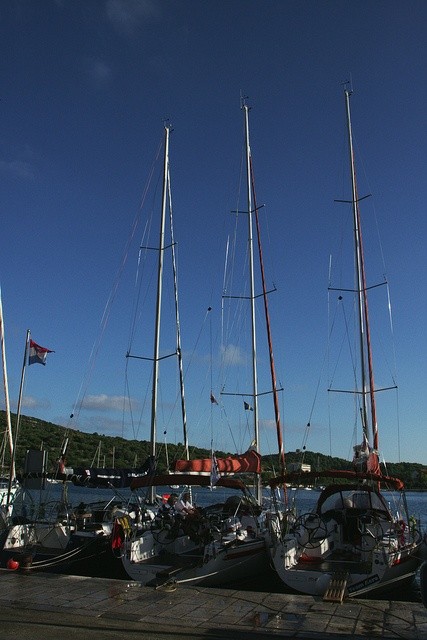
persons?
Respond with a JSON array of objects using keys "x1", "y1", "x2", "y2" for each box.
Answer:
[
  {"x1": 177, "y1": 492, "x2": 194, "y2": 516},
  {"x1": 156, "y1": 495, "x2": 175, "y2": 525}
]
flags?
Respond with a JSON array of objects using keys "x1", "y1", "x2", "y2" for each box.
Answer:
[{"x1": 28, "y1": 341, "x2": 54, "y2": 367}]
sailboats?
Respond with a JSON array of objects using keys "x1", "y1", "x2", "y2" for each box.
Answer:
[
  {"x1": 269, "y1": 71, "x2": 427, "y2": 598},
  {"x1": 0, "y1": 295, "x2": 25, "y2": 517},
  {"x1": 112, "y1": 90, "x2": 298, "y2": 586},
  {"x1": 0, "y1": 117, "x2": 193, "y2": 570}
]
